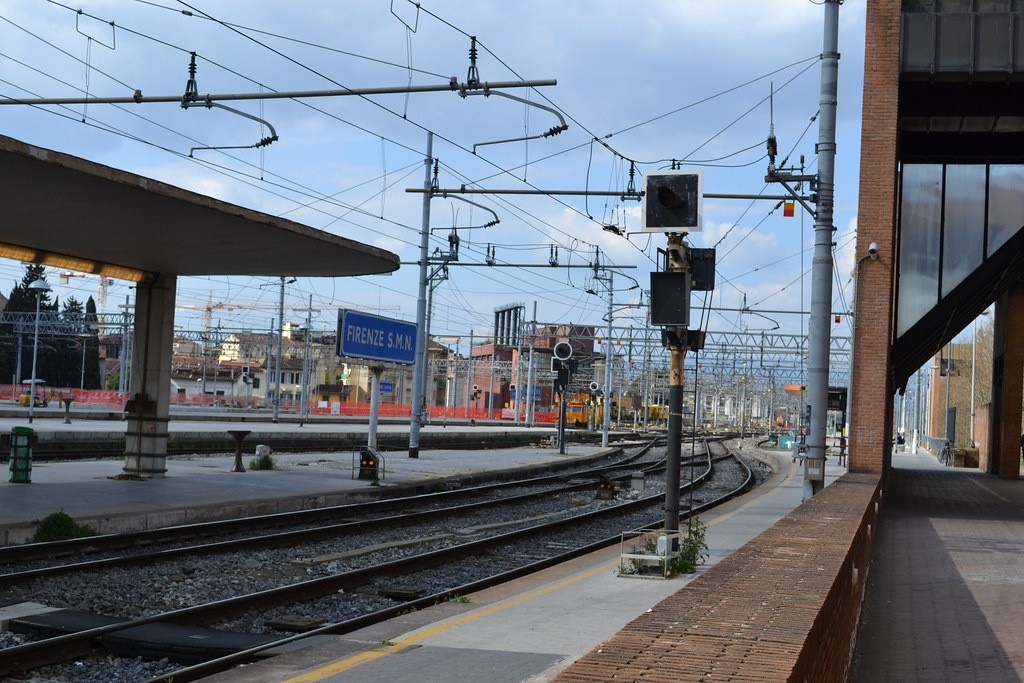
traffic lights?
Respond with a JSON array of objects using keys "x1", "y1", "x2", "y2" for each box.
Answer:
[{"x1": 645, "y1": 172, "x2": 702, "y2": 231}]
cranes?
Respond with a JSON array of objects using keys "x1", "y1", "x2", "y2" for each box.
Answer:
[
  {"x1": 60, "y1": 269, "x2": 116, "y2": 335},
  {"x1": 179, "y1": 288, "x2": 400, "y2": 351}
]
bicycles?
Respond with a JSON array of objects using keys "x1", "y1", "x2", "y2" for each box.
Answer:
[{"x1": 937, "y1": 439, "x2": 953, "y2": 467}]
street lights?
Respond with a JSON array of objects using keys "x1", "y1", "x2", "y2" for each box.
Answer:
[{"x1": 26, "y1": 271, "x2": 51, "y2": 422}]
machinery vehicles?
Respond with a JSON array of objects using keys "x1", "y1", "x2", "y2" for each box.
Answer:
[{"x1": 19, "y1": 378, "x2": 61, "y2": 407}]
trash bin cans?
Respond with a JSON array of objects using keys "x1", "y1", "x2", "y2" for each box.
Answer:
[{"x1": 8, "y1": 426, "x2": 39, "y2": 484}]
